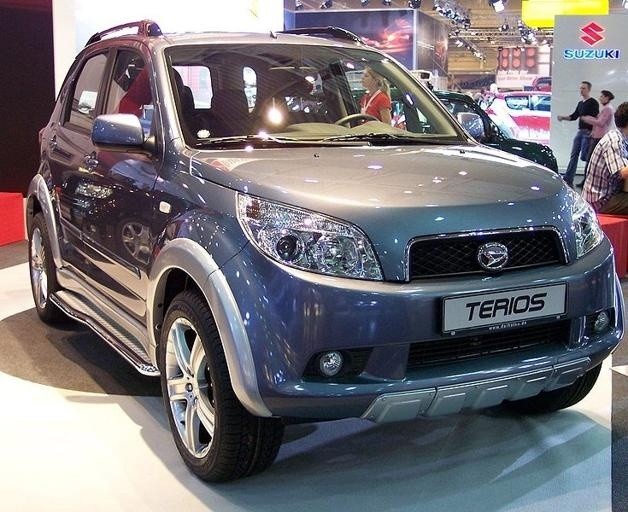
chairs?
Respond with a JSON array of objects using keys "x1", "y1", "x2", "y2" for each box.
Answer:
[{"x1": 175, "y1": 68, "x2": 329, "y2": 135}]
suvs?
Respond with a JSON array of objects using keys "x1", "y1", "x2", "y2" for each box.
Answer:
[{"x1": 23, "y1": 15, "x2": 628, "y2": 485}]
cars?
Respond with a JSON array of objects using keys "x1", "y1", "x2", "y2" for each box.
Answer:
[
  {"x1": 477, "y1": 88, "x2": 552, "y2": 144},
  {"x1": 346, "y1": 84, "x2": 561, "y2": 175}
]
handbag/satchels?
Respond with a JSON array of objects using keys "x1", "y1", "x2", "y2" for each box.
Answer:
[{"x1": 580, "y1": 135, "x2": 590, "y2": 161}]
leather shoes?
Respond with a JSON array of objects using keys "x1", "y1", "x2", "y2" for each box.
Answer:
[{"x1": 576, "y1": 179, "x2": 585, "y2": 188}]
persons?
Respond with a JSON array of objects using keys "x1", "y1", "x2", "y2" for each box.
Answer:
[
  {"x1": 360, "y1": 66, "x2": 391, "y2": 127},
  {"x1": 557, "y1": 81, "x2": 615, "y2": 187},
  {"x1": 472, "y1": 87, "x2": 486, "y2": 102},
  {"x1": 581, "y1": 102, "x2": 628, "y2": 214}
]
